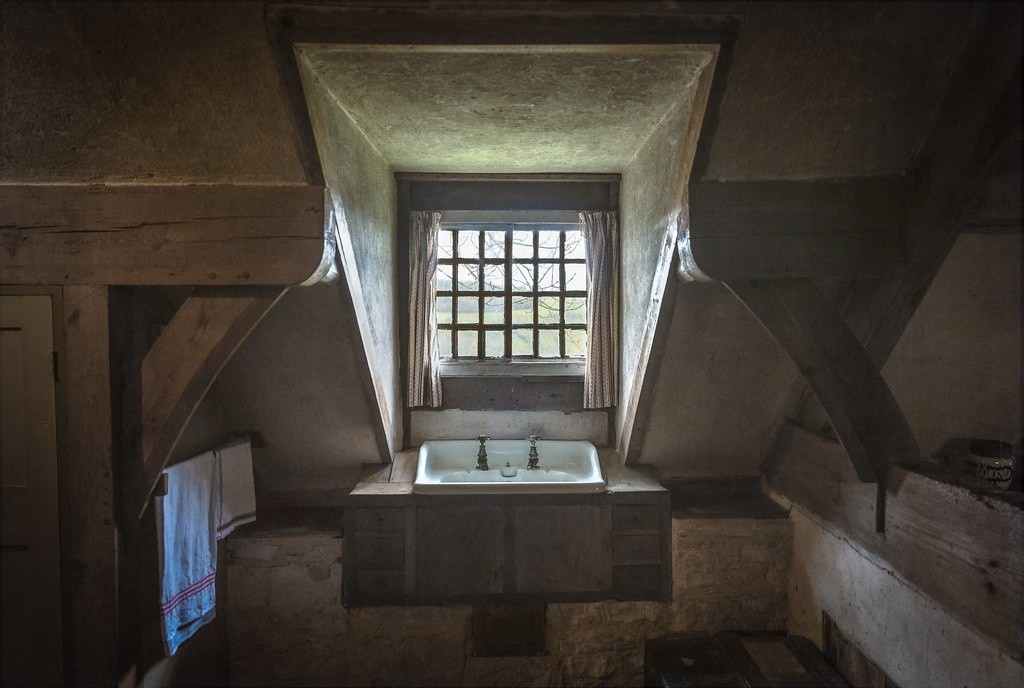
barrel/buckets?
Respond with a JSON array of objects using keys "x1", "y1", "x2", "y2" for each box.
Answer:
[{"x1": 965, "y1": 439, "x2": 1016, "y2": 492}]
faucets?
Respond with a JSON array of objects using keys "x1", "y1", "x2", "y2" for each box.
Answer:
[
  {"x1": 476, "y1": 435, "x2": 490, "y2": 470},
  {"x1": 526, "y1": 434, "x2": 541, "y2": 469}
]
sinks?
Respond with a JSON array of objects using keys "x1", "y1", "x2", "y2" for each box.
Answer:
[{"x1": 413, "y1": 438, "x2": 606, "y2": 494}]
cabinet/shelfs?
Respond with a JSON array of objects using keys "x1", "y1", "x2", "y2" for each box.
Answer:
[{"x1": 338, "y1": 464, "x2": 675, "y2": 607}]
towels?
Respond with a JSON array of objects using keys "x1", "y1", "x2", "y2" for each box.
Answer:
[
  {"x1": 154, "y1": 449, "x2": 218, "y2": 658},
  {"x1": 212, "y1": 437, "x2": 257, "y2": 541}
]
